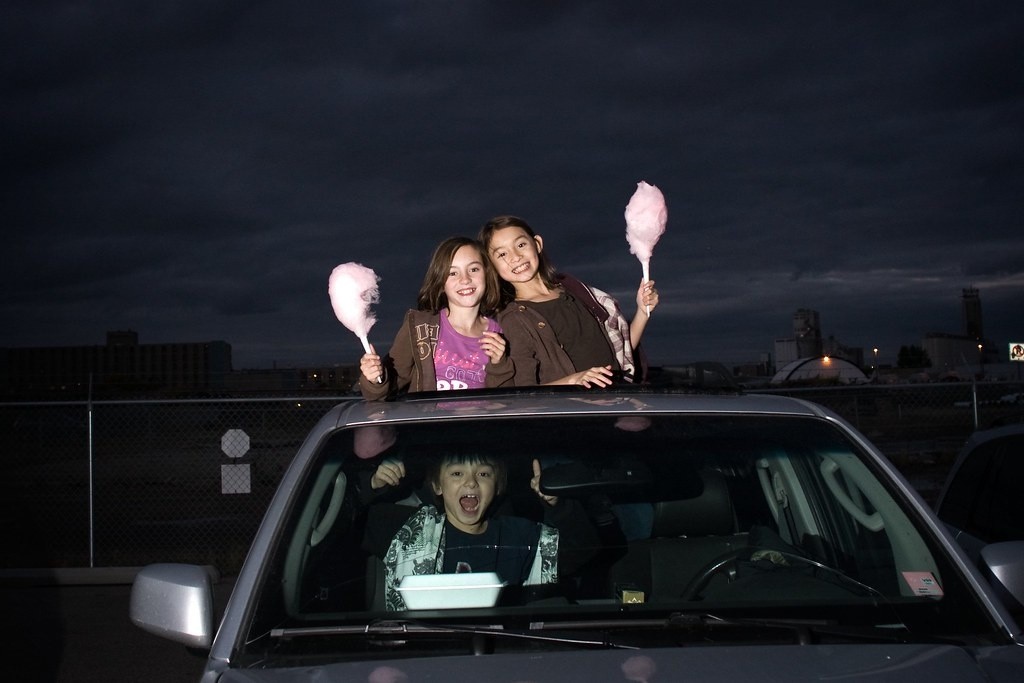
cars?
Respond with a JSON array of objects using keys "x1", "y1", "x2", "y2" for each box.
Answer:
[
  {"x1": 931, "y1": 418, "x2": 1023, "y2": 545},
  {"x1": 124, "y1": 381, "x2": 1024, "y2": 683}
]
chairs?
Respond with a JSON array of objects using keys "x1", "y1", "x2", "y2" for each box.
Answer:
[
  {"x1": 368, "y1": 449, "x2": 515, "y2": 611},
  {"x1": 604, "y1": 477, "x2": 829, "y2": 601}
]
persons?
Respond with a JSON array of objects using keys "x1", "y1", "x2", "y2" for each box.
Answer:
[
  {"x1": 478, "y1": 216, "x2": 656, "y2": 484},
  {"x1": 350, "y1": 432, "x2": 602, "y2": 615},
  {"x1": 357, "y1": 236, "x2": 517, "y2": 458}
]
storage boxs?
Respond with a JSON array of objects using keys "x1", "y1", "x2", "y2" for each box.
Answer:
[{"x1": 389, "y1": 574, "x2": 508, "y2": 610}]
